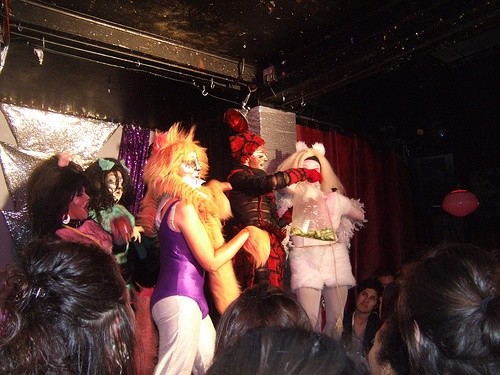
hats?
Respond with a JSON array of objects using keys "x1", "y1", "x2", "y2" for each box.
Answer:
[{"x1": 229, "y1": 129, "x2": 265, "y2": 165}]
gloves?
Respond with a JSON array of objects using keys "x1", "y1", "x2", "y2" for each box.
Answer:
[{"x1": 283, "y1": 168, "x2": 323, "y2": 186}]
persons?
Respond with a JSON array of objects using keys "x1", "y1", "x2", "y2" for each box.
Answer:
[
  {"x1": 0, "y1": 124, "x2": 500, "y2": 375},
  {"x1": 274, "y1": 141, "x2": 368, "y2": 339}
]
